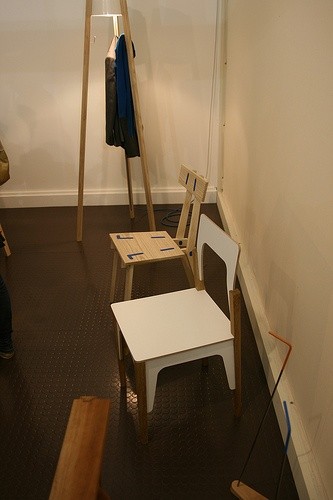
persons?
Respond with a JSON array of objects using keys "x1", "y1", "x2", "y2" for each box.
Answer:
[{"x1": 0, "y1": 140, "x2": 19, "y2": 358}]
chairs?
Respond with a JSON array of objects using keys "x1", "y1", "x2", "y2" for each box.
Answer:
[
  {"x1": 107, "y1": 212, "x2": 244, "y2": 440},
  {"x1": 105, "y1": 163, "x2": 209, "y2": 302}
]
